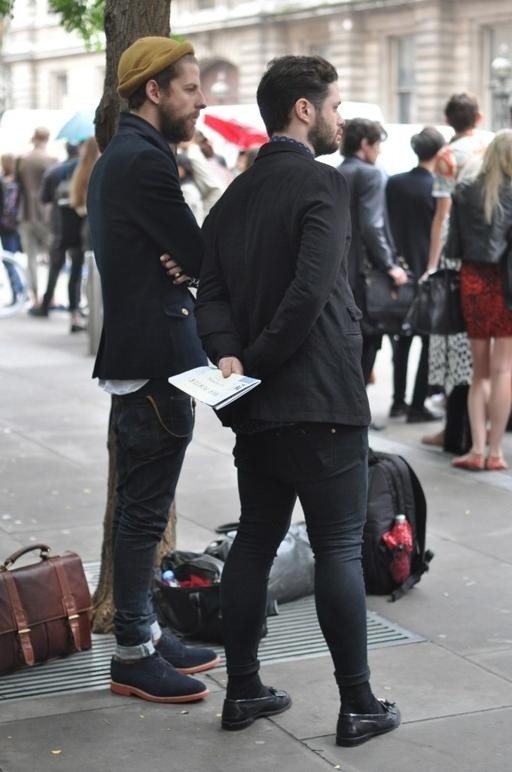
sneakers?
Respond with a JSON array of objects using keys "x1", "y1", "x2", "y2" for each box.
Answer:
[
  {"x1": 110, "y1": 633, "x2": 220, "y2": 704},
  {"x1": 452, "y1": 453, "x2": 508, "y2": 469}
]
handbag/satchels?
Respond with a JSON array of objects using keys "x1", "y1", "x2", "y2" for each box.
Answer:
[
  {"x1": 1, "y1": 544, "x2": 93, "y2": 677},
  {"x1": 443, "y1": 386, "x2": 472, "y2": 454},
  {"x1": 152, "y1": 521, "x2": 315, "y2": 643},
  {"x1": 361, "y1": 452, "x2": 427, "y2": 595},
  {"x1": 356, "y1": 270, "x2": 466, "y2": 334}
]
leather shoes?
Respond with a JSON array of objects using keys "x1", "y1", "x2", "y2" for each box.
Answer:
[
  {"x1": 336, "y1": 700, "x2": 400, "y2": 747},
  {"x1": 221, "y1": 688, "x2": 291, "y2": 730}
]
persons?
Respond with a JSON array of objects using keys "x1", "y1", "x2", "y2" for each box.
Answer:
[
  {"x1": 194, "y1": 57, "x2": 400, "y2": 748},
  {"x1": 0, "y1": 92, "x2": 261, "y2": 334},
  {"x1": 335, "y1": 92, "x2": 512, "y2": 471},
  {"x1": 87, "y1": 37, "x2": 219, "y2": 705}
]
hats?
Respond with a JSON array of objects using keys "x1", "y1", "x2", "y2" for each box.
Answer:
[{"x1": 116, "y1": 36, "x2": 193, "y2": 98}]
